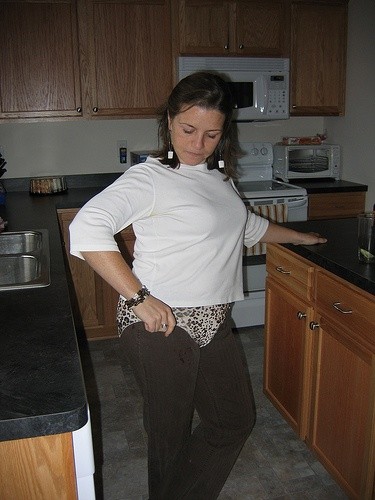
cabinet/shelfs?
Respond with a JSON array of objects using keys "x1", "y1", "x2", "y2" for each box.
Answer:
[
  {"x1": 308, "y1": 192, "x2": 365, "y2": 221},
  {"x1": 57, "y1": 208, "x2": 136, "y2": 342},
  {"x1": 0, "y1": 0, "x2": 349, "y2": 124},
  {"x1": 262, "y1": 242, "x2": 375, "y2": 500}
]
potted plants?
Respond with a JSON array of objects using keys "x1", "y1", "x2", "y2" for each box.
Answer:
[{"x1": 0, "y1": 152, "x2": 8, "y2": 205}]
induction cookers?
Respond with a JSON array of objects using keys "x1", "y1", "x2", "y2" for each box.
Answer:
[{"x1": 230, "y1": 141, "x2": 307, "y2": 200}]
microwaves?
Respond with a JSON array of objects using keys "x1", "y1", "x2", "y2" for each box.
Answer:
[{"x1": 176, "y1": 55, "x2": 290, "y2": 122}]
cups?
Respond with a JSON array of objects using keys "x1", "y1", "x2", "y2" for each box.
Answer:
[{"x1": 357, "y1": 213, "x2": 375, "y2": 264}]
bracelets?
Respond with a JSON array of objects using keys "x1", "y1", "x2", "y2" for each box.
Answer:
[{"x1": 124, "y1": 285, "x2": 151, "y2": 310}]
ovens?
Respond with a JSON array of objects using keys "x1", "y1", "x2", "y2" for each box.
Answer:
[
  {"x1": 272, "y1": 142, "x2": 341, "y2": 182},
  {"x1": 230, "y1": 195, "x2": 310, "y2": 330}
]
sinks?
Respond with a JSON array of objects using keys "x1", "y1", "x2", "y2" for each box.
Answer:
[
  {"x1": 0, "y1": 228, "x2": 50, "y2": 256},
  {"x1": 0, "y1": 254, "x2": 50, "y2": 291}
]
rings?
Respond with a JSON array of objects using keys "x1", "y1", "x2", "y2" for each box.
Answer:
[{"x1": 160, "y1": 323, "x2": 169, "y2": 329}]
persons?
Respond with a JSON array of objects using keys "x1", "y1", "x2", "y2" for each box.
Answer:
[{"x1": 68, "y1": 70, "x2": 327, "y2": 500}]
